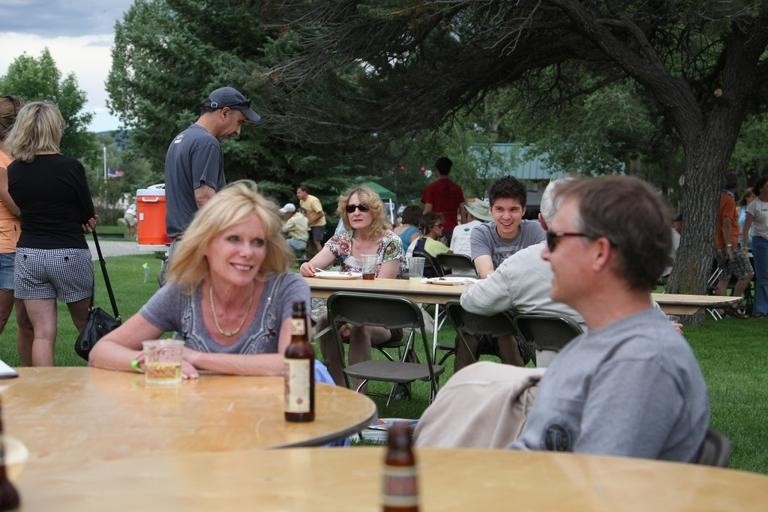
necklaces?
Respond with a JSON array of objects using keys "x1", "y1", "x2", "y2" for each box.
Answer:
[{"x1": 206, "y1": 281, "x2": 255, "y2": 339}]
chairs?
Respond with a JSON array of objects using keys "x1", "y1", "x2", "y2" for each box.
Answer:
[
  {"x1": 341, "y1": 273, "x2": 427, "y2": 409},
  {"x1": 327, "y1": 292, "x2": 445, "y2": 411},
  {"x1": 404, "y1": 250, "x2": 581, "y2": 369},
  {"x1": 708, "y1": 262, "x2": 731, "y2": 320}
]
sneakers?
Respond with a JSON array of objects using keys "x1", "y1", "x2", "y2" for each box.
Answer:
[{"x1": 725, "y1": 305, "x2": 749, "y2": 319}]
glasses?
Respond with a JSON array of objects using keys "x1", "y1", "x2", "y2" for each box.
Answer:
[
  {"x1": 345, "y1": 203, "x2": 369, "y2": 213},
  {"x1": 432, "y1": 223, "x2": 441, "y2": 229},
  {"x1": 545, "y1": 230, "x2": 618, "y2": 253}
]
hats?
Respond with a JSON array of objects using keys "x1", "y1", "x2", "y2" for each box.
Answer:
[
  {"x1": 279, "y1": 203, "x2": 295, "y2": 213},
  {"x1": 463, "y1": 201, "x2": 492, "y2": 221},
  {"x1": 202, "y1": 86, "x2": 261, "y2": 122}
]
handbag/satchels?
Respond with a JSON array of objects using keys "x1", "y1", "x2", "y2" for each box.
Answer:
[{"x1": 74, "y1": 307, "x2": 121, "y2": 362}]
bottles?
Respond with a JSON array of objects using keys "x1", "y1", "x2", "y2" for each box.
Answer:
[
  {"x1": 1, "y1": 398, "x2": 22, "y2": 511},
  {"x1": 382, "y1": 419, "x2": 419, "y2": 511},
  {"x1": 282, "y1": 299, "x2": 315, "y2": 423},
  {"x1": 142, "y1": 262, "x2": 151, "y2": 285}
]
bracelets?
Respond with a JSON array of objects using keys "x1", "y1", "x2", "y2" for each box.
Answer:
[{"x1": 131, "y1": 350, "x2": 145, "y2": 375}]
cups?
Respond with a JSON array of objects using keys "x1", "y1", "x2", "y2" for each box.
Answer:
[
  {"x1": 408, "y1": 256, "x2": 425, "y2": 282},
  {"x1": 141, "y1": 338, "x2": 186, "y2": 388},
  {"x1": 361, "y1": 253, "x2": 379, "y2": 279}
]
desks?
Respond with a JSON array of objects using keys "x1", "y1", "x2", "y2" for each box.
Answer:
[
  {"x1": 299, "y1": 271, "x2": 741, "y2": 411},
  {"x1": 0, "y1": 365, "x2": 375, "y2": 448},
  {"x1": 0, "y1": 443, "x2": 768, "y2": 512}
]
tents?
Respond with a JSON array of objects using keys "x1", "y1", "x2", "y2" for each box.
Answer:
[{"x1": 341, "y1": 175, "x2": 396, "y2": 229}]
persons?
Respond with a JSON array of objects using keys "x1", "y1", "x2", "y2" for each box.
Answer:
[
  {"x1": 1, "y1": 95, "x2": 35, "y2": 367},
  {"x1": 459, "y1": 177, "x2": 684, "y2": 368},
  {"x1": 86, "y1": 178, "x2": 341, "y2": 395},
  {"x1": 162, "y1": 85, "x2": 262, "y2": 286},
  {"x1": 656, "y1": 173, "x2": 767, "y2": 320},
  {"x1": 454, "y1": 174, "x2": 548, "y2": 373},
  {"x1": 3, "y1": 96, "x2": 99, "y2": 368},
  {"x1": 278, "y1": 185, "x2": 327, "y2": 267},
  {"x1": 300, "y1": 186, "x2": 409, "y2": 396},
  {"x1": 500, "y1": 171, "x2": 713, "y2": 471},
  {"x1": 392, "y1": 157, "x2": 494, "y2": 276}
]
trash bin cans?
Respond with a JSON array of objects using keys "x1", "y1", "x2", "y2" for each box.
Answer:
[{"x1": 135, "y1": 188, "x2": 174, "y2": 245}]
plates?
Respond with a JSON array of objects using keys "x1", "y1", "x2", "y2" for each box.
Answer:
[
  {"x1": 312, "y1": 271, "x2": 362, "y2": 279},
  {"x1": 423, "y1": 276, "x2": 476, "y2": 287}
]
